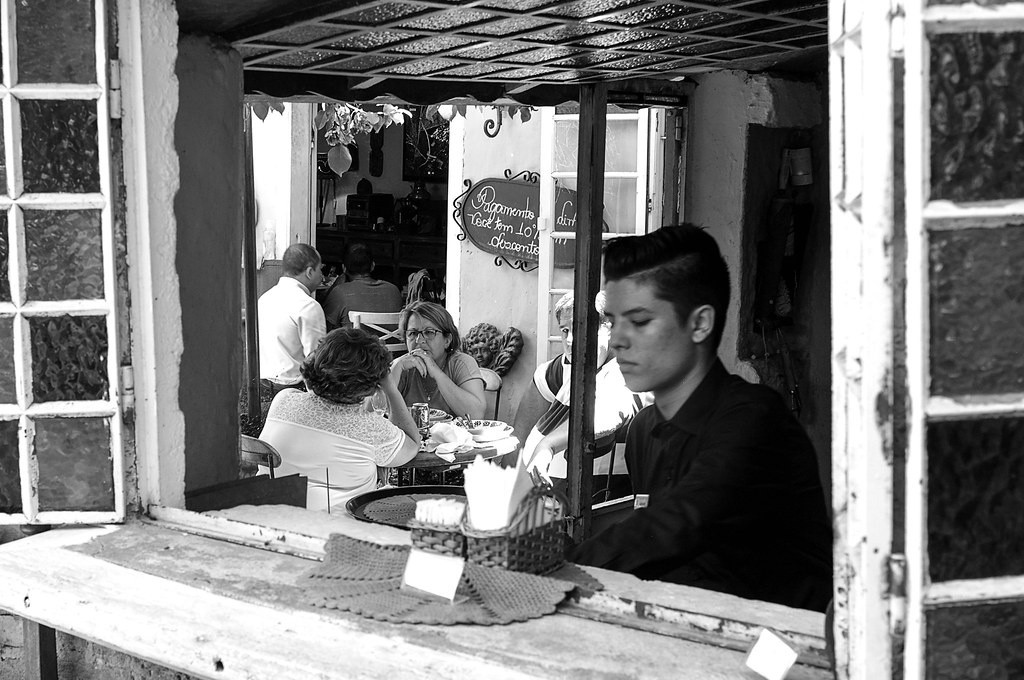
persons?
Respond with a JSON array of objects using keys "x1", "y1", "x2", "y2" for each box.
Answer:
[
  {"x1": 260, "y1": 326, "x2": 421, "y2": 509},
  {"x1": 499, "y1": 286, "x2": 653, "y2": 492},
  {"x1": 323, "y1": 249, "x2": 402, "y2": 332},
  {"x1": 527, "y1": 222, "x2": 832, "y2": 615},
  {"x1": 363, "y1": 300, "x2": 486, "y2": 423},
  {"x1": 257, "y1": 242, "x2": 327, "y2": 412}
]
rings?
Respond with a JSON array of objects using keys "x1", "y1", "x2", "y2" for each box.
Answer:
[{"x1": 423, "y1": 352, "x2": 426, "y2": 354}]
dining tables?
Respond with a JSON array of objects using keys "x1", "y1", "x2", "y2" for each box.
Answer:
[
  {"x1": 395, "y1": 434, "x2": 520, "y2": 486},
  {"x1": 315, "y1": 275, "x2": 338, "y2": 290}
]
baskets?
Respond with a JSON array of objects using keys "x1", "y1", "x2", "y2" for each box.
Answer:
[{"x1": 405, "y1": 465, "x2": 565, "y2": 577}]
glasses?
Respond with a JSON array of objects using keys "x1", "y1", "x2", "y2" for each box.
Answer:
[{"x1": 404, "y1": 329, "x2": 448, "y2": 340}]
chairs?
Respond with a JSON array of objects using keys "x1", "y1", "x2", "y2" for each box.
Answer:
[
  {"x1": 479, "y1": 368, "x2": 502, "y2": 421},
  {"x1": 241, "y1": 433, "x2": 281, "y2": 480},
  {"x1": 347, "y1": 310, "x2": 408, "y2": 351},
  {"x1": 564, "y1": 432, "x2": 618, "y2": 506}
]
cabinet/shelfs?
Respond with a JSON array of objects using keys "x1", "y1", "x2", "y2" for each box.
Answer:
[{"x1": 315, "y1": 226, "x2": 447, "y2": 295}]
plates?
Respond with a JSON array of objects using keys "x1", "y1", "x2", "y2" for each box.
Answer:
[
  {"x1": 451, "y1": 420, "x2": 515, "y2": 441},
  {"x1": 409, "y1": 408, "x2": 453, "y2": 423}
]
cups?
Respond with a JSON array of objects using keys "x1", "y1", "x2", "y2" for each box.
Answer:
[{"x1": 364, "y1": 390, "x2": 387, "y2": 415}]
